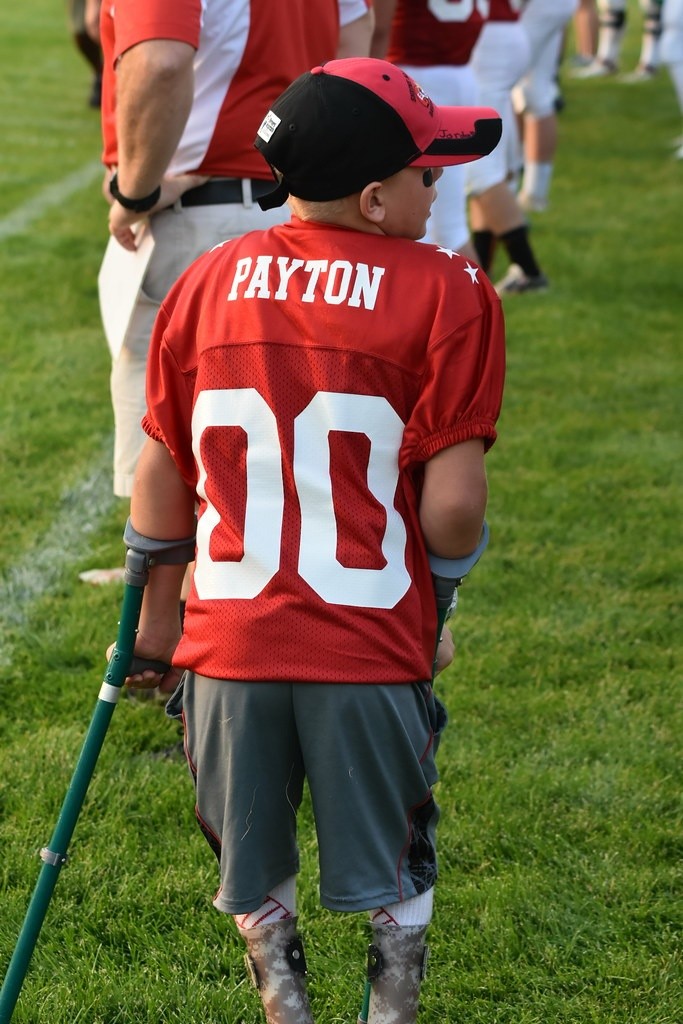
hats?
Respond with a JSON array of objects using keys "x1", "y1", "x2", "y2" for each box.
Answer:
[{"x1": 253, "y1": 58, "x2": 503, "y2": 215}]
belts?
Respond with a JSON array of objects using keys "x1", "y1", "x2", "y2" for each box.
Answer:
[{"x1": 164, "y1": 178, "x2": 278, "y2": 209}]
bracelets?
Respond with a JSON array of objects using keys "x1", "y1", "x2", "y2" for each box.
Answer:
[{"x1": 110, "y1": 170, "x2": 161, "y2": 212}]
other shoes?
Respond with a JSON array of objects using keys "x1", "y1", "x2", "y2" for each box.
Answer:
[
  {"x1": 623, "y1": 64, "x2": 657, "y2": 82},
  {"x1": 572, "y1": 56, "x2": 617, "y2": 77}
]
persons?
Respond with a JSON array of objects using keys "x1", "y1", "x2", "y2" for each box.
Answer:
[
  {"x1": 70, "y1": 1, "x2": 682, "y2": 502},
  {"x1": 104, "y1": 54, "x2": 508, "y2": 1024}
]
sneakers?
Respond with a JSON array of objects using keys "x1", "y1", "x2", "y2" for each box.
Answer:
[{"x1": 494, "y1": 263, "x2": 550, "y2": 296}]
